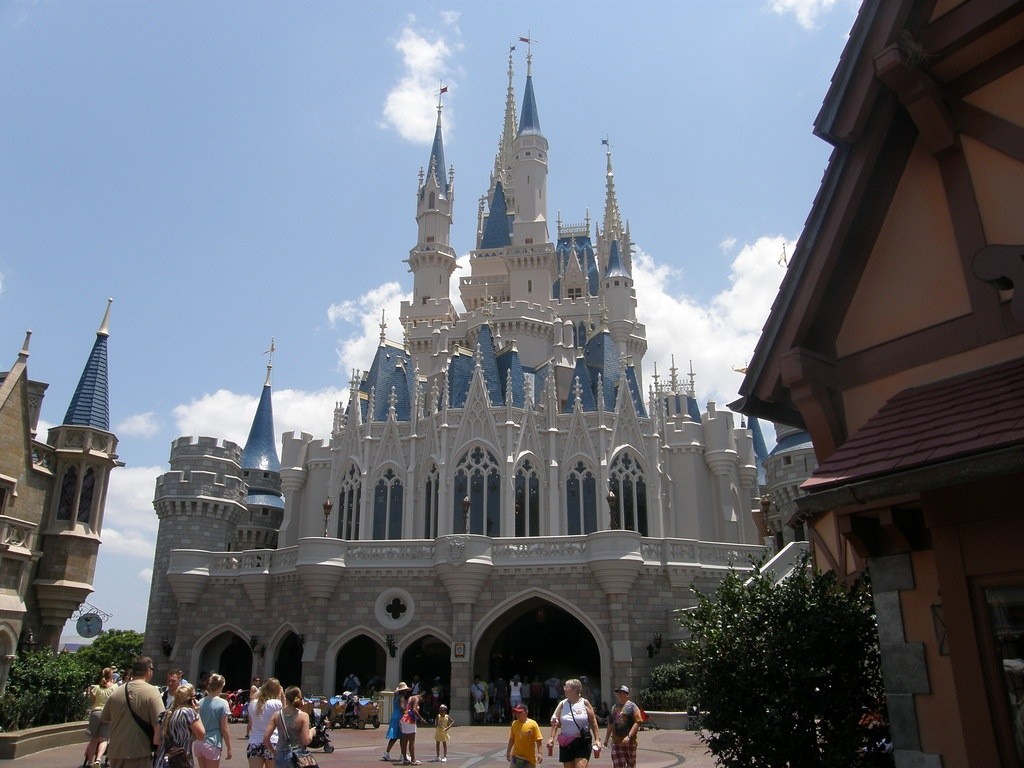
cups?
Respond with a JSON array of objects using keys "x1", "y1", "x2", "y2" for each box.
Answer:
[
  {"x1": 548, "y1": 744, "x2": 553, "y2": 755},
  {"x1": 592, "y1": 745, "x2": 600, "y2": 758}
]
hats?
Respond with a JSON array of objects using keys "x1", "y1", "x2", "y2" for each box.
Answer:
[
  {"x1": 512, "y1": 704, "x2": 528, "y2": 713},
  {"x1": 394, "y1": 682, "x2": 412, "y2": 693},
  {"x1": 110, "y1": 666, "x2": 118, "y2": 673},
  {"x1": 614, "y1": 685, "x2": 629, "y2": 694}
]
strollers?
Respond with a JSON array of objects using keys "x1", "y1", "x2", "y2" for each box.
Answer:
[{"x1": 310, "y1": 710, "x2": 335, "y2": 753}]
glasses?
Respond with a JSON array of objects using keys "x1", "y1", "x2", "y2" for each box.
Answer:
[{"x1": 255, "y1": 680, "x2": 260, "y2": 682}]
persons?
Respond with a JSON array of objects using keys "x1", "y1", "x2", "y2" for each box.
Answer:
[
  {"x1": 88, "y1": 666, "x2": 133, "y2": 768},
  {"x1": 264, "y1": 686, "x2": 316, "y2": 768},
  {"x1": 246, "y1": 678, "x2": 286, "y2": 768},
  {"x1": 548, "y1": 679, "x2": 602, "y2": 768},
  {"x1": 507, "y1": 704, "x2": 544, "y2": 768},
  {"x1": 603, "y1": 684, "x2": 643, "y2": 768},
  {"x1": 382, "y1": 682, "x2": 411, "y2": 760},
  {"x1": 432, "y1": 704, "x2": 454, "y2": 762},
  {"x1": 399, "y1": 690, "x2": 428, "y2": 765},
  {"x1": 471, "y1": 674, "x2": 595, "y2": 724},
  {"x1": 101, "y1": 656, "x2": 166, "y2": 768},
  {"x1": 194, "y1": 673, "x2": 232, "y2": 768},
  {"x1": 343, "y1": 669, "x2": 360, "y2": 694},
  {"x1": 595, "y1": 702, "x2": 609, "y2": 724},
  {"x1": 410, "y1": 675, "x2": 443, "y2": 723},
  {"x1": 161, "y1": 668, "x2": 188, "y2": 710},
  {"x1": 366, "y1": 672, "x2": 374, "y2": 695},
  {"x1": 154, "y1": 684, "x2": 205, "y2": 768},
  {"x1": 244, "y1": 676, "x2": 261, "y2": 740}
]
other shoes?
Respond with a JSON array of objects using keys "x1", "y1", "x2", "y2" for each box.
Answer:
[
  {"x1": 382, "y1": 751, "x2": 391, "y2": 761},
  {"x1": 430, "y1": 756, "x2": 441, "y2": 762},
  {"x1": 399, "y1": 754, "x2": 409, "y2": 761},
  {"x1": 442, "y1": 757, "x2": 446, "y2": 762},
  {"x1": 245, "y1": 735, "x2": 249, "y2": 739},
  {"x1": 87, "y1": 764, "x2": 93, "y2": 768},
  {"x1": 403, "y1": 759, "x2": 411, "y2": 765},
  {"x1": 94, "y1": 762, "x2": 100, "y2": 768},
  {"x1": 411, "y1": 761, "x2": 421, "y2": 765}
]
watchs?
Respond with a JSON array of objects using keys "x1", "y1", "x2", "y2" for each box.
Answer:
[{"x1": 628, "y1": 734, "x2": 633, "y2": 738}]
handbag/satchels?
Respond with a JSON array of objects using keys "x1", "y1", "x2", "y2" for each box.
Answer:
[
  {"x1": 133, "y1": 714, "x2": 158, "y2": 753},
  {"x1": 580, "y1": 728, "x2": 592, "y2": 744},
  {"x1": 292, "y1": 752, "x2": 318, "y2": 768},
  {"x1": 474, "y1": 700, "x2": 485, "y2": 713},
  {"x1": 409, "y1": 707, "x2": 418, "y2": 722},
  {"x1": 165, "y1": 747, "x2": 191, "y2": 768},
  {"x1": 556, "y1": 733, "x2": 576, "y2": 747}
]
